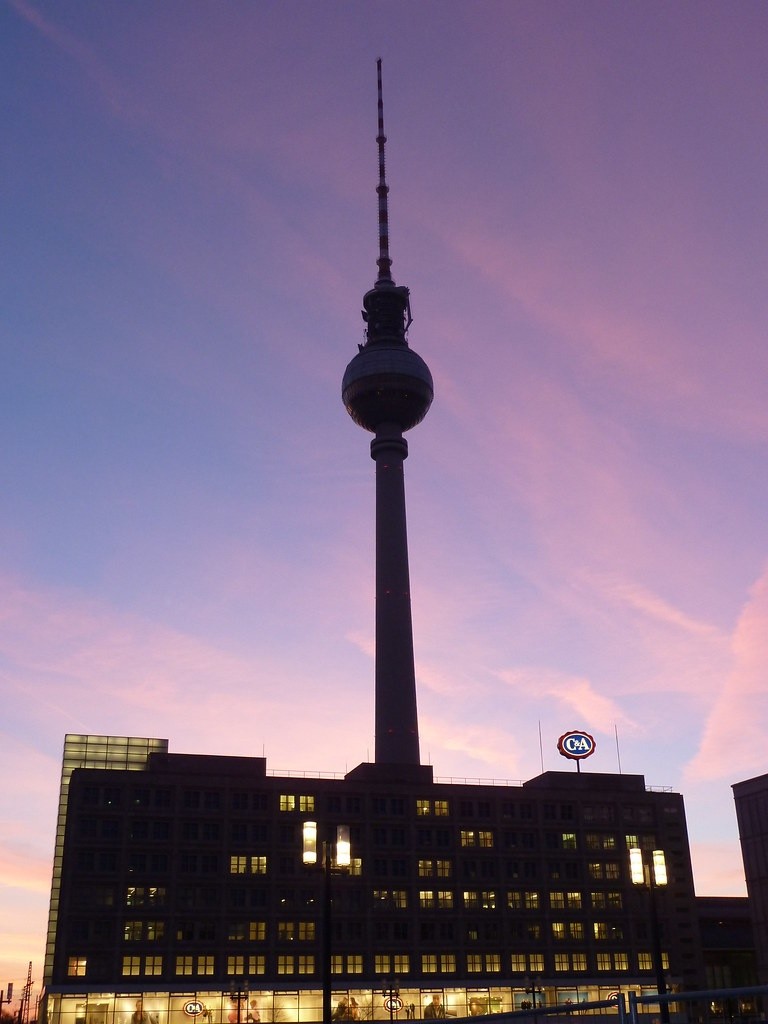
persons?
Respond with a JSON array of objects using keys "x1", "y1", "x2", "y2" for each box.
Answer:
[
  {"x1": 423, "y1": 993, "x2": 447, "y2": 1019},
  {"x1": 581, "y1": 998, "x2": 587, "y2": 1014},
  {"x1": 405, "y1": 1003, "x2": 415, "y2": 1019},
  {"x1": 129, "y1": 1000, "x2": 158, "y2": 1023},
  {"x1": 332, "y1": 996, "x2": 363, "y2": 1021},
  {"x1": 244, "y1": 1000, "x2": 261, "y2": 1024},
  {"x1": 565, "y1": 998, "x2": 573, "y2": 1014}
]
